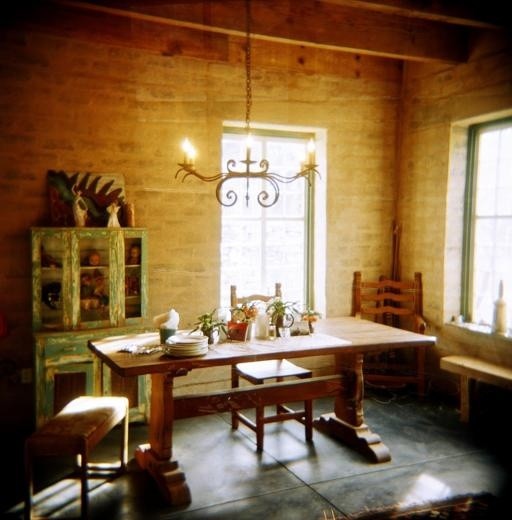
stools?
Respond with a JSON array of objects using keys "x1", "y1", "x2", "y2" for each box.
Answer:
[{"x1": 21, "y1": 391, "x2": 133, "y2": 519}]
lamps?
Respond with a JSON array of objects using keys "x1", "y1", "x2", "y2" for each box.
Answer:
[{"x1": 168, "y1": 1, "x2": 324, "y2": 209}]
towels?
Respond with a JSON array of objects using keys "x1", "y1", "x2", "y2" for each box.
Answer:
[{"x1": 154, "y1": 308, "x2": 181, "y2": 329}]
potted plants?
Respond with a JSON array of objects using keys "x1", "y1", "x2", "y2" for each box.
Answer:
[
  {"x1": 227, "y1": 301, "x2": 258, "y2": 342},
  {"x1": 188, "y1": 308, "x2": 230, "y2": 345},
  {"x1": 266, "y1": 299, "x2": 298, "y2": 336}
]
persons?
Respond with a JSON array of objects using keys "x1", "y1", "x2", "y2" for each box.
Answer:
[
  {"x1": 105, "y1": 200, "x2": 122, "y2": 228},
  {"x1": 71, "y1": 183, "x2": 89, "y2": 228}
]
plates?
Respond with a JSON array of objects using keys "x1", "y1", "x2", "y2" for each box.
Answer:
[{"x1": 165, "y1": 334, "x2": 209, "y2": 358}]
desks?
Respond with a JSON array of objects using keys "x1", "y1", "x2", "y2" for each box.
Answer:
[{"x1": 83, "y1": 315, "x2": 438, "y2": 514}]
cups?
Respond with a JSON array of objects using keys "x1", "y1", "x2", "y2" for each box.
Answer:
[{"x1": 158, "y1": 328, "x2": 176, "y2": 343}]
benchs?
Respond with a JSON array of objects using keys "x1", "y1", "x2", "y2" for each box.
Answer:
[{"x1": 437, "y1": 350, "x2": 511, "y2": 420}]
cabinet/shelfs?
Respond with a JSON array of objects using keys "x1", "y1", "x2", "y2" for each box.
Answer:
[{"x1": 29, "y1": 223, "x2": 152, "y2": 429}]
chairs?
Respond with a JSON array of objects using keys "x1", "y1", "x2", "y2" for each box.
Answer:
[
  {"x1": 347, "y1": 270, "x2": 428, "y2": 406},
  {"x1": 229, "y1": 281, "x2": 315, "y2": 454}
]
